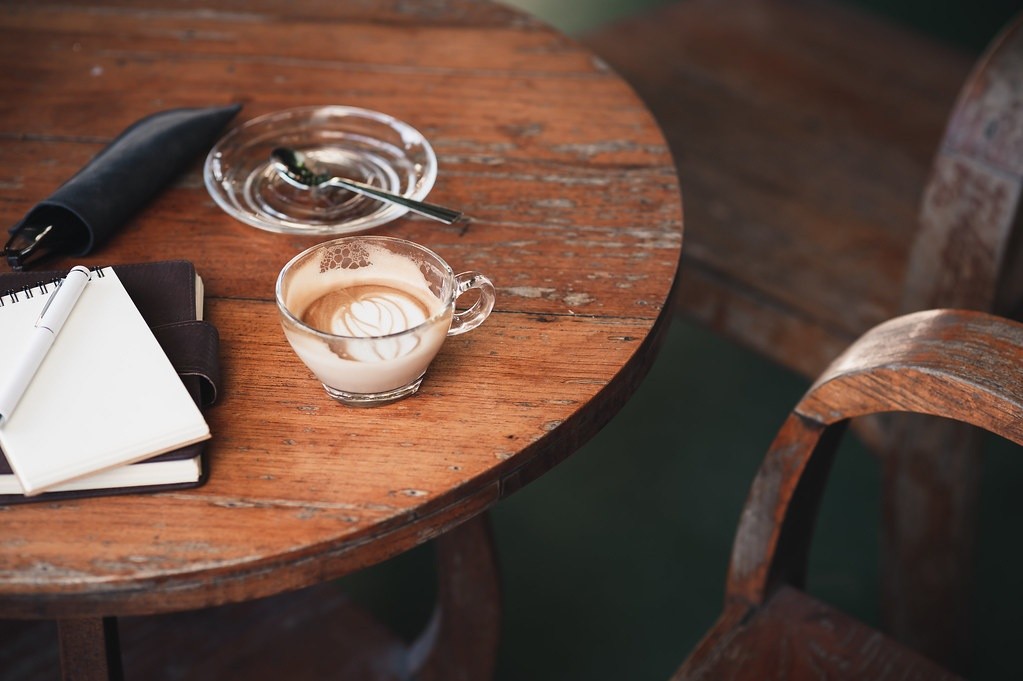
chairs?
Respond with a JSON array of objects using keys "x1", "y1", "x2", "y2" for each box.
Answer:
[
  {"x1": 669, "y1": 310, "x2": 1023, "y2": 681},
  {"x1": 571, "y1": 0, "x2": 1023, "y2": 482}
]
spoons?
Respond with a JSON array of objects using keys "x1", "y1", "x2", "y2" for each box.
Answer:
[{"x1": 270, "y1": 146, "x2": 463, "y2": 227}]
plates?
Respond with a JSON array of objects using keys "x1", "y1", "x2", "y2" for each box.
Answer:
[{"x1": 204, "y1": 105, "x2": 438, "y2": 235}]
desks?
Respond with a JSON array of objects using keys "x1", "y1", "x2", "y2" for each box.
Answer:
[{"x1": 0, "y1": 0, "x2": 687, "y2": 681}]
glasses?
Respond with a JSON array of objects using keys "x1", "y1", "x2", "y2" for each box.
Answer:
[{"x1": 0, "y1": 207, "x2": 80, "y2": 273}]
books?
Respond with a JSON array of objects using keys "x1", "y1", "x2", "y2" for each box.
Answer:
[{"x1": 0, "y1": 258, "x2": 221, "y2": 505}]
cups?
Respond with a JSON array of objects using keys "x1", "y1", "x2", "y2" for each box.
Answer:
[{"x1": 277, "y1": 235, "x2": 496, "y2": 409}]
